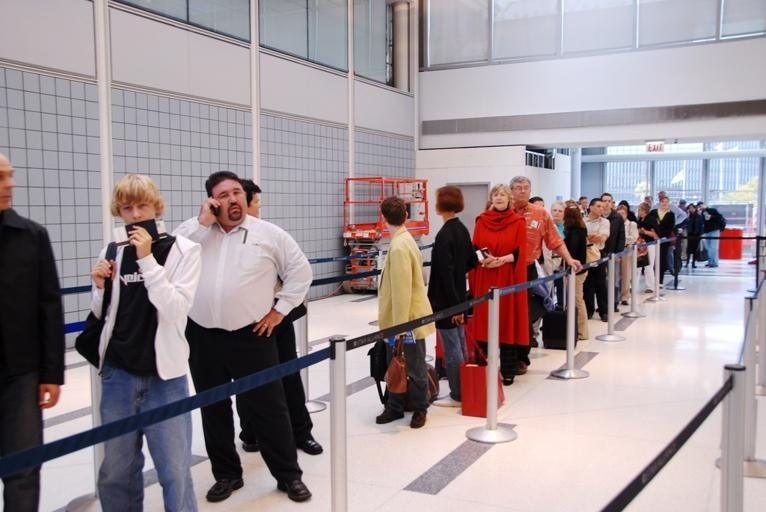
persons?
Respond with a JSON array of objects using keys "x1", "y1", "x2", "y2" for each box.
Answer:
[
  {"x1": 373, "y1": 194, "x2": 437, "y2": 430},
  {"x1": 427, "y1": 182, "x2": 481, "y2": 408},
  {"x1": 73, "y1": 172, "x2": 204, "y2": 511},
  {"x1": 171, "y1": 170, "x2": 313, "y2": 502},
  {"x1": 236, "y1": 174, "x2": 327, "y2": 456},
  {"x1": 508, "y1": 176, "x2": 721, "y2": 375},
  {"x1": 468, "y1": 183, "x2": 531, "y2": 387}
]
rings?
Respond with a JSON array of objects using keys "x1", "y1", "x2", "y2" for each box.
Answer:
[{"x1": 263, "y1": 322, "x2": 268, "y2": 327}]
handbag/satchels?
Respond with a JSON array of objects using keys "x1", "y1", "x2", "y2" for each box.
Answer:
[
  {"x1": 694, "y1": 246, "x2": 710, "y2": 261},
  {"x1": 461, "y1": 353, "x2": 505, "y2": 419},
  {"x1": 382, "y1": 350, "x2": 410, "y2": 395},
  {"x1": 393, "y1": 357, "x2": 442, "y2": 410},
  {"x1": 74, "y1": 310, "x2": 104, "y2": 372}
]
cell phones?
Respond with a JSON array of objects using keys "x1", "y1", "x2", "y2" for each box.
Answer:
[{"x1": 211, "y1": 205, "x2": 220, "y2": 216}]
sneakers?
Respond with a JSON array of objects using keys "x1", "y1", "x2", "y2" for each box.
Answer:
[
  {"x1": 430, "y1": 391, "x2": 469, "y2": 409},
  {"x1": 375, "y1": 404, "x2": 404, "y2": 424},
  {"x1": 410, "y1": 408, "x2": 427, "y2": 429},
  {"x1": 502, "y1": 358, "x2": 529, "y2": 385},
  {"x1": 584, "y1": 295, "x2": 630, "y2": 321}
]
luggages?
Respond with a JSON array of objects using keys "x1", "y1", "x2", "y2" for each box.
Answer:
[{"x1": 541, "y1": 304, "x2": 580, "y2": 351}]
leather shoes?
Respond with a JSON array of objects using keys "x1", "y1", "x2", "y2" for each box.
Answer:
[
  {"x1": 205, "y1": 472, "x2": 245, "y2": 503},
  {"x1": 242, "y1": 434, "x2": 260, "y2": 453},
  {"x1": 294, "y1": 434, "x2": 325, "y2": 454},
  {"x1": 276, "y1": 476, "x2": 313, "y2": 503}
]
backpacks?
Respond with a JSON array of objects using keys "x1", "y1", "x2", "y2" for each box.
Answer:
[{"x1": 720, "y1": 216, "x2": 726, "y2": 231}]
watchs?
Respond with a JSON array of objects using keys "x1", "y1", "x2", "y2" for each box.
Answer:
[{"x1": 0, "y1": 152, "x2": 68, "y2": 512}]
